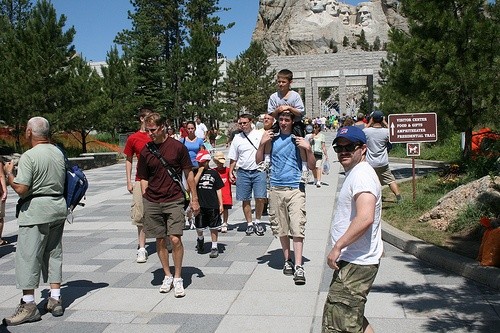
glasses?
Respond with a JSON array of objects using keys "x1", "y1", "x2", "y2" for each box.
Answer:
[
  {"x1": 332, "y1": 143, "x2": 360, "y2": 153},
  {"x1": 238, "y1": 120, "x2": 250, "y2": 125}
]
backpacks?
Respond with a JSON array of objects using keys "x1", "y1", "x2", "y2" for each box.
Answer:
[{"x1": 53, "y1": 144, "x2": 88, "y2": 216}]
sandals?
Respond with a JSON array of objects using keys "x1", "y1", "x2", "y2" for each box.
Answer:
[{"x1": 0, "y1": 239, "x2": 9, "y2": 246}]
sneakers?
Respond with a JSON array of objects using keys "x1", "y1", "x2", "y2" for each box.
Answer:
[
  {"x1": 159, "y1": 275, "x2": 173, "y2": 293},
  {"x1": 283, "y1": 260, "x2": 295, "y2": 276},
  {"x1": 2, "y1": 299, "x2": 42, "y2": 326},
  {"x1": 173, "y1": 277, "x2": 185, "y2": 298},
  {"x1": 293, "y1": 265, "x2": 306, "y2": 284},
  {"x1": 45, "y1": 296, "x2": 63, "y2": 317}
]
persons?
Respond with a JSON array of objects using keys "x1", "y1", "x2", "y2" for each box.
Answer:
[
  {"x1": 0, "y1": 120, "x2": 9, "y2": 245},
  {"x1": 126, "y1": 69, "x2": 402, "y2": 298},
  {"x1": 1, "y1": 116, "x2": 68, "y2": 325},
  {"x1": 320, "y1": 125, "x2": 384, "y2": 333}
]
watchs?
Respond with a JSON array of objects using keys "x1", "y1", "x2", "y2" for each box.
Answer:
[{"x1": 7, "y1": 172, "x2": 12, "y2": 176}]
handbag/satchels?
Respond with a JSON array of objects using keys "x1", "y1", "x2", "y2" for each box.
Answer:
[
  {"x1": 314, "y1": 151, "x2": 323, "y2": 159},
  {"x1": 322, "y1": 156, "x2": 331, "y2": 175}
]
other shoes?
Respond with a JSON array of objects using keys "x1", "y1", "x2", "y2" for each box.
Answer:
[
  {"x1": 136, "y1": 248, "x2": 149, "y2": 263},
  {"x1": 255, "y1": 222, "x2": 265, "y2": 236},
  {"x1": 299, "y1": 171, "x2": 309, "y2": 184},
  {"x1": 188, "y1": 219, "x2": 196, "y2": 230},
  {"x1": 196, "y1": 237, "x2": 205, "y2": 252},
  {"x1": 246, "y1": 224, "x2": 254, "y2": 236},
  {"x1": 221, "y1": 223, "x2": 229, "y2": 233},
  {"x1": 209, "y1": 248, "x2": 220, "y2": 258},
  {"x1": 313, "y1": 178, "x2": 321, "y2": 187},
  {"x1": 257, "y1": 160, "x2": 272, "y2": 172}
]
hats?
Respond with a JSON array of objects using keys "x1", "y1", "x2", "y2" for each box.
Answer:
[
  {"x1": 372, "y1": 111, "x2": 383, "y2": 119},
  {"x1": 195, "y1": 150, "x2": 211, "y2": 163},
  {"x1": 213, "y1": 151, "x2": 227, "y2": 164},
  {"x1": 332, "y1": 126, "x2": 367, "y2": 144}
]
